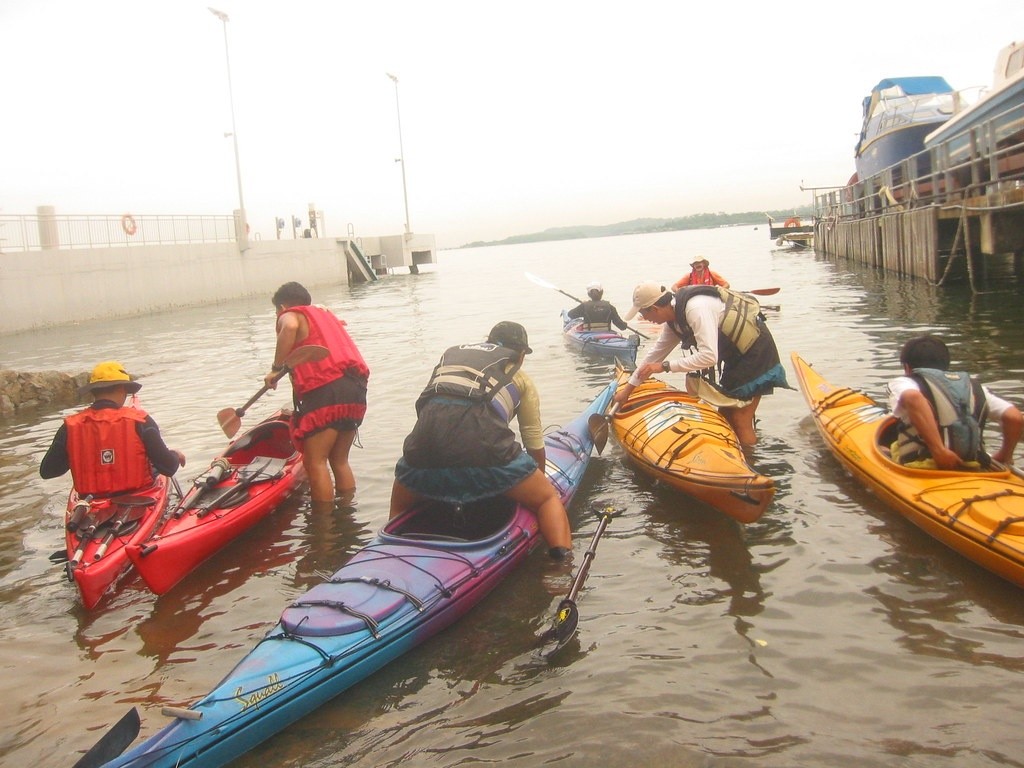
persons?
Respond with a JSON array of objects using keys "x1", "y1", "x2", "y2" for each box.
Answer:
[
  {"x1": 671, "y1": 255, "x2": 730, "y2": 292},
  {"x1": 40, "y1": 361, "x2": 185, "y2": 525},
  {"x1": 610, "y1": 283, "x2": 780, "y2": 444},
  {"x1": 389, "y1": 321, "x2": 572, "y2": 555},
  {"x1": 887, "y1": 336, "x2": 1024, "y2": 470},
  {"x1": 567, "y1": 283, "x2": 627, "y2": 330},
  {"x1": 264, "y1": 282, "x2": 369, "y2": 500}
]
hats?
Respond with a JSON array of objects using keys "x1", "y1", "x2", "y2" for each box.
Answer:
[
  {"x1": 486, "y1": 321, "x2": 532, "y2": 354},
  {"x1": 689, "y1": 256, "x2": 709, "y2": 267},
  {"x1": 624, "y1": 282, "x2": 669, "y2": 321},
  {"x1": 75, "y1": 362, "x2": 142, "y2": 394},
  {"x1": 586, "y1": 283, "x2": 601, "y2": 294}
]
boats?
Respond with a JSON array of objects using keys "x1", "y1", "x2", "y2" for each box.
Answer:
[
  {"x1": 767, "y1": 215, "x2": 814, "y2": 241},
  {"x1": 69, "y1": 382, "x2": 617, "y2": 768},
  {"x1": 788, "y1": 350, "x2": 1024, "y2": 589},
  {"x1": 923, "y1": 41, "x2": 1024, "y2": 194},
  {"x1": 852, "y1": 76, "x2": 972, "y2": 216},
  {"x1": 123, "y1": 399, "x2": 308, "y2": 595},
  {"x1": 605, "y1": 355, "x2": 777, "y2": 526},
  {"x1": 557, "y1": 308, "x2": 640, "y2": 362},
  {"x1": 63, "y1": 395, "x2": 171, "y2": 612}
]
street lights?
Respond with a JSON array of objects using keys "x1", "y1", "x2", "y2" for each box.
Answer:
[
  {"x1": 395, "y1": 158, "x2": 410, "y2": 235},
  {"x1": 224, "y1": 131, "x2": 243, "y2": 210}
]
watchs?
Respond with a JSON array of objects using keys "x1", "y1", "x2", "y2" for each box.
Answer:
[{"x1": 662, "y1": 361, "x2": 669, "y2": 371}]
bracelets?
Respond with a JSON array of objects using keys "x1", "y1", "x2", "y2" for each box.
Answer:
[{"x1": 271, "y1": 365, "x2": 282, "y2": 371}]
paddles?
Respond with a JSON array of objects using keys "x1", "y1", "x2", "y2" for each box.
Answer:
[
  {"x1": 543, "y1": 504, "x2": 626, "y2": 657},
  {"x1": 174, "y1": 449, "x2": 285, "y2": 519},
  {"x1": 739, "y1": 285, "x2": 780, "y2": 295},
  {"x1": 68, "y1": 490, "x2": 156, "y2": 567},
  {"x1": 526, "y1": 270, "x2": 650, "y2": 339},
  {"x1": 586, "y1": 402, "x2": 622, "y2": 458},
  {"x1": 216, "y1": 345, "x2": 331, "y2": 440}
]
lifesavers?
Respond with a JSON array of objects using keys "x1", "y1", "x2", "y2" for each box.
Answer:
[
  {"x1": 122, "y1": 213, "x2": 136, "y2": 235},
  {"x1": 783, "y1": 218, "x2": 800, "y2": 228}
]
